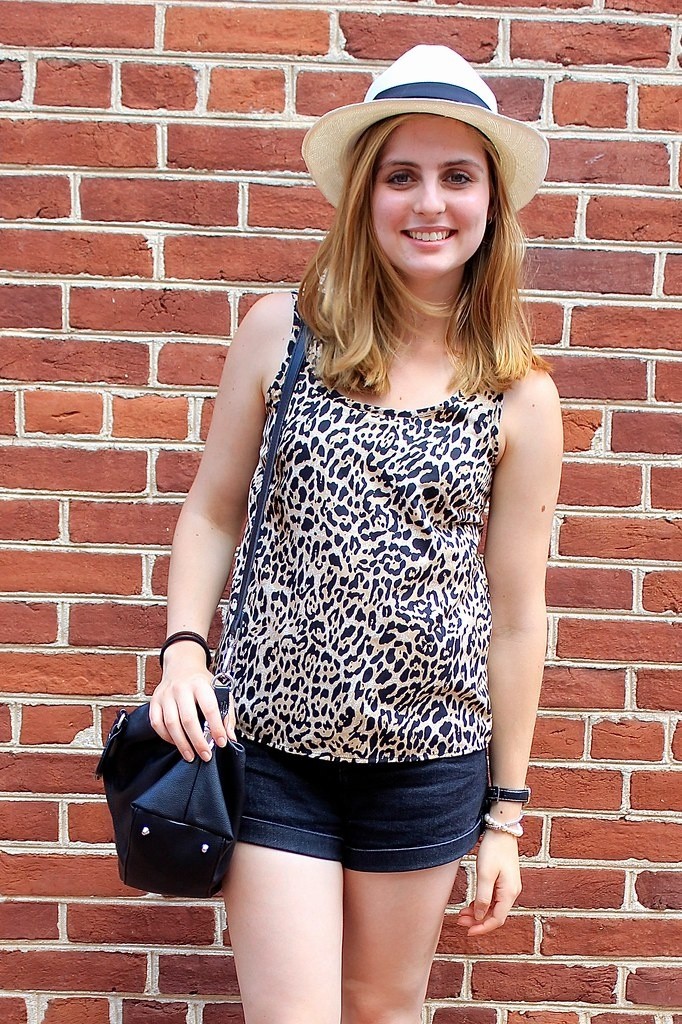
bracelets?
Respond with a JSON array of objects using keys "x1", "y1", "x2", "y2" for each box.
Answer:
[
  {"x1": 160, "y1": 631, "x2": 211, "y2": 670},
  {"x1": 483, "y1": 812, "x2": 524, "y2": 838}
]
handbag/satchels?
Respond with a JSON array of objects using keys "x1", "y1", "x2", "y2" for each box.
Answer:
[{"x1": 95, "y1": 701, "x2": 247, "y2": 898}]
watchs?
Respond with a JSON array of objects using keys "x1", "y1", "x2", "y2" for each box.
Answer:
[{"x1": 486, "y1": 785, "x2": 532, "y2": 808}]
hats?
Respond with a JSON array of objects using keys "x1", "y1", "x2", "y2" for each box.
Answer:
[{"x1": 300, "y1": 43, "x2": 550, "y2": 213}]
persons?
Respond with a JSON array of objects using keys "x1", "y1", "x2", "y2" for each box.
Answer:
[{"x1": 149, "y1": 43, "x2": 562, "y2": 1024}]
